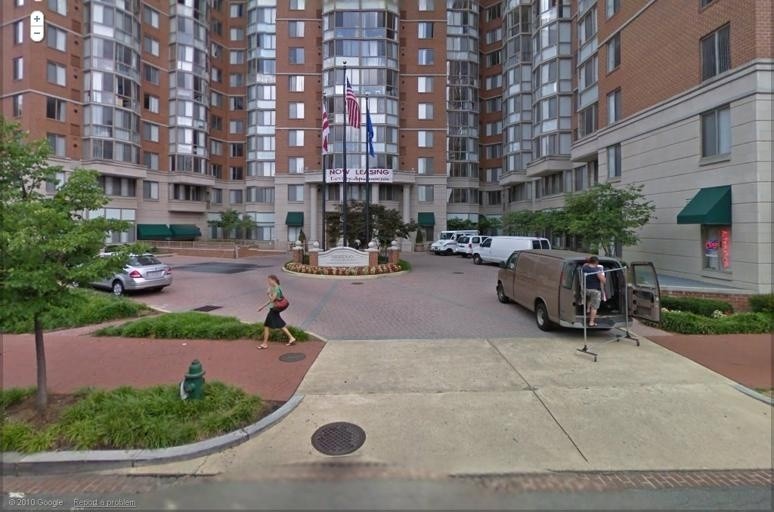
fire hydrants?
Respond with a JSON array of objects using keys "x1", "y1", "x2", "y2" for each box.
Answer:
[{"x1": 179, "y1": 358, "x2": 207, "y2": 400}]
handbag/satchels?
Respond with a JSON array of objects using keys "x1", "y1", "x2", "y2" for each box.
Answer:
[{"x1": 273, "y1": 296, "x2": 289, "y2": 312}]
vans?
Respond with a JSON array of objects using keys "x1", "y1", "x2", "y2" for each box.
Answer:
[
  {"x1": 472, "y1": 235, "x2": 552, "y2": 268},
  {"x1": 495, "y1": 247, "x2": 661, "y2": 333},
  {"x1": 430, "y1": 230, "x2": 479, "y2": 257},
  {"x1": 455, "y1": 235, "x2": 488, "y2": 258}
]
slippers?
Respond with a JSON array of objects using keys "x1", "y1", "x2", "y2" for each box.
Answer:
[{"x1": 255, "y1": 337, "x2": 296, "y2": 350}]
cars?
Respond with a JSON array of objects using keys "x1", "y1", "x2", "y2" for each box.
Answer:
[{"x1": 61, "y1": 251, "x2": 174, "y2": 298}]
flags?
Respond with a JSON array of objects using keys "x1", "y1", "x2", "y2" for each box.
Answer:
[
  {"x1": 319, "y1": 103, "x2": 329, "y2": 155},
  {"x1": 366, "y1": 108, "x2": 375, "y2": 159},
  {"x1": 345, "y1": 78, "x2": 361, "y2": 130}
]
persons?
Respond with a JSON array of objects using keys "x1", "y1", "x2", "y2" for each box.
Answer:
[
  {"x1": 255, "y1": 274, "x2": 296, "y2": 349},
  {"x1": 580, "y1": 256, "x2": 609, "y2": 328}
]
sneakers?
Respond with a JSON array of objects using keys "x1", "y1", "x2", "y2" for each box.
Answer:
[{"x1": 586, "y1": 310, "x2": 598, "y2": 327}]
